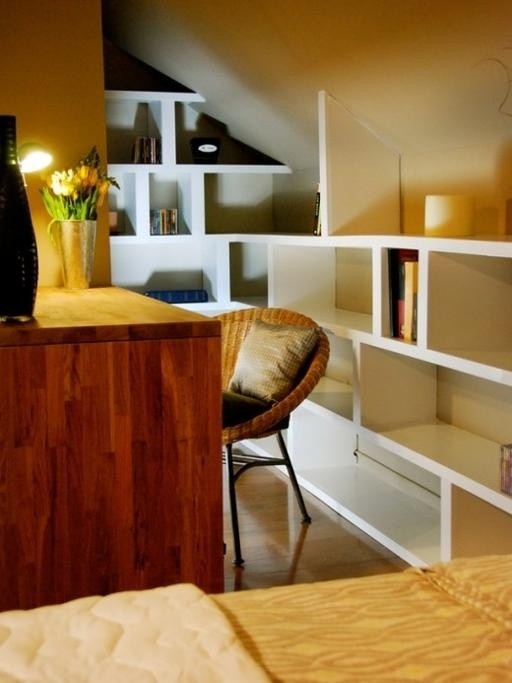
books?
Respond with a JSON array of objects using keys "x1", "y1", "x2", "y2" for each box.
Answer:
[
  {"x1": 313, "y1": 183, "x2": 321, "y2": 235},
  {"x1": 388, "y1": 250, "x2": 419, "y2": 341},
  {"x1": 150, "y1": 209, "x2": 178, "y2": 236},
  {"x1": 134, "y1": 136, "x2": 161, "y2": 164},
  {"x1": 500, "y1": 444, "x2": 511, "y2": 498}
]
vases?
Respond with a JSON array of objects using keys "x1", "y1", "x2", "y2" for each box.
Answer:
[{"x1": 52, "y1": 218, "x2": 97, "y2": 291}]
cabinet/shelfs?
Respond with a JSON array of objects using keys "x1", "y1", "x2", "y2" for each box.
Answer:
[{"x1": 103, "y1": 89, "x2": 512, "y2": 572}]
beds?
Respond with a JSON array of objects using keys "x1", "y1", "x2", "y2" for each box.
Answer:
[{"x1": 1, "y1": 553, "x2": 512, "y2": 683}]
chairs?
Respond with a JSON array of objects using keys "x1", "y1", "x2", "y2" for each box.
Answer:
[{"x1": 201, "y1": 308, "x2": 329, "y2": 567}]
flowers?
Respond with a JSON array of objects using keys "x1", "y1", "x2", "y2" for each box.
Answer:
[{"x1": 36, "y1": 144, "x2": 123, "y2": 221}]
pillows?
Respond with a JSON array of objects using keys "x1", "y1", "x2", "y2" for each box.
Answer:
[{"x1": 226, "y1": 316, "x2": 321, "y2": 404}]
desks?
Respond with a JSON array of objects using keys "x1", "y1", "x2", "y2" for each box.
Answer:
[{"x1": 4, "y1": 283, "x2": 224, "y2": 608}]
